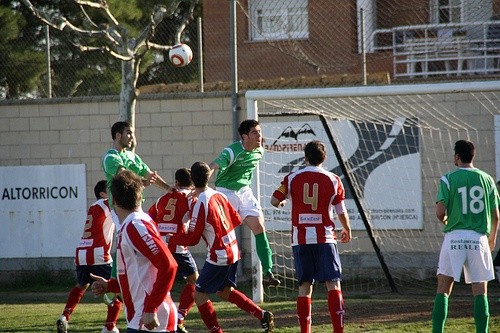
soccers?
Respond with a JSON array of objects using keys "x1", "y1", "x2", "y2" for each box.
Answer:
[{"x1": 169, "y1": 44, "x2": 193, "y2": 67}]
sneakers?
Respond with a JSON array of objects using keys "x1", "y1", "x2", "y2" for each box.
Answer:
[
  {"x1": 102, "y1": 326, "x2": 119, "y2": 333},
  {"x1": 57, "y1": 315, "x2": 68, "y2": 333},
  {"x1": 263, "y1": 273, "x2": 280, "y2": 287},
  {"x1": 177, "y1": 324, "x2": 188, "y2": 333},
  {"x1": 261, "y1": 311, "x2": 274, "y2": 333}
]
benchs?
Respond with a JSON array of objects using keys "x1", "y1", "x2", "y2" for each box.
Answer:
[{"x1": 405, "y1": 36, "x2": 474, "y2": 78}]
